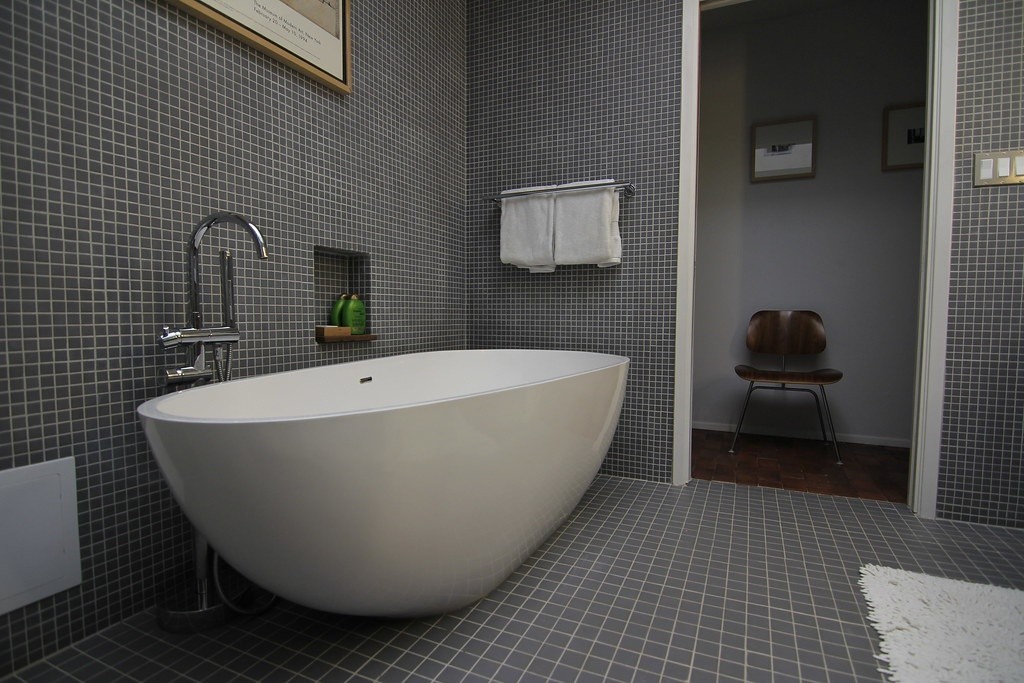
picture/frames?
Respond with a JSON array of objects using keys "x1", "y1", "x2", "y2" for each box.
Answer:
[
  {"x1": 168, "y1": 0, "x2": 352, "y2": 96},
  {"x1": 749, "y1": 117, "x2": 817, "y2": 182},
  {"x1": 881, "y1": 103, "x2": 925, "y2": 172}
]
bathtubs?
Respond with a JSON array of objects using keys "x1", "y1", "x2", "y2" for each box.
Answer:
[{"x1": 132, "y1": 347, "x2": 632, "y2": 621}]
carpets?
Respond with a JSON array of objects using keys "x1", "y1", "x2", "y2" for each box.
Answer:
[
  {"x1": 858, "y1": 564, "x2": 1024, "y2": 683},
  {"x1": 692, "y1": 428, "x2": 911, "y2": 503}
]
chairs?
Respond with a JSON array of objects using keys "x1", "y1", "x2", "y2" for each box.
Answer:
[{"x1": 728, "y1": 308, "x2": 844, "y2": 466}]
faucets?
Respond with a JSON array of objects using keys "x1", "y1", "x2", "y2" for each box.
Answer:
[{"x1": 186, "y1": 210, "x2": 270, "y2": 382}]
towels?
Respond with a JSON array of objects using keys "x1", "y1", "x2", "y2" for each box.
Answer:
[{"x1": 498, "y1": 178, "x2": 624, "y2": 275}]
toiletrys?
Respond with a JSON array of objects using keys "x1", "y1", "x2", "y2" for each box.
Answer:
[{"x1": 331, "y1": 292, "x2": 367, "y2": 335}]
